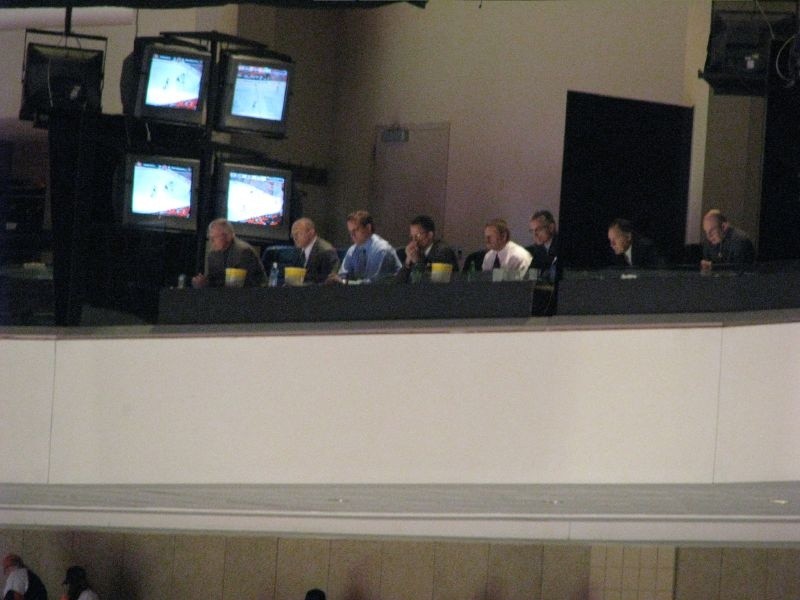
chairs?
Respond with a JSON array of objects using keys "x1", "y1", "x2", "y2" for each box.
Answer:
[{"x1": 259, "y1": 239, "x2": 773, "y2": 279}]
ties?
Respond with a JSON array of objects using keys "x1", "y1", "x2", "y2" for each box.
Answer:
[
  {"x1": 491, "y1": 253, "x2": 500, "y2": 270},
  {"x1": 299, "y1": 251, "x2": 306, "y2": 268},
  {"x1": 358, "y1": 249, "x2": 366, "y2": 280}
]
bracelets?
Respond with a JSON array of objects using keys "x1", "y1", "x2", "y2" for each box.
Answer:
[{"x1": 341, "y1": 279, "x2": 347, "y2": 286}]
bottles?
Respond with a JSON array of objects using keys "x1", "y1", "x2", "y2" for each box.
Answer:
[{"x1": 268, "y1": 262, "x2": 279, "y2": 288}]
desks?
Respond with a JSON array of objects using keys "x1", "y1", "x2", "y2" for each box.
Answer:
[
  {"x1": 557, "y1": 262, "x2": 800, "y2": 312},
  {"x1": 159, "y1": 272, "x2": 552, "y2": 329}
]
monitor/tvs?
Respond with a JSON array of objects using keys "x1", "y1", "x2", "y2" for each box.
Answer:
[
  {"x1": 701, "y1": 13, "x2": 800, "y2": 98},
  {"x1": 215, "y1": 54, "x2": 292, "y2": 135},
  {"x1": 19, "y1": 45, "x2": 104, "y2": 120},
  {"x1": 123, "y1": 153, "x2": 200, "y2": 232},
  {"x1": 133, "y1": 44, "x2": 211, "y2": 125},
  {"x1": 216, "y1": 163, "x2": 292, "y2": 241}
]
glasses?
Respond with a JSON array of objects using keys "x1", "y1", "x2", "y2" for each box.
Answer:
[{"x1": 410, "y1": 231, "x2": 426, "y2": 240}]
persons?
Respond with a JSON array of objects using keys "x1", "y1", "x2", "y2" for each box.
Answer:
[
  {"x1": 481, "y1": 210, "x2": 583, "y2": 279},
  {"x1": 0, "y1": 554, "x2": 48, "y2": 600},
  {"x1": 304, "y1": 589, "x2": 326, "y2": 600},
  {"x1": 191, "y1": 210, "x2": 460, "y2": 289},
  {"x1": 60, "y1": 566, "x2": 100, "y2": 600},
  {"x1": 700, "y1": 209, "x2": 757, "y2": 271},
  {"x1": 607, "y1": 218, "x2": 665, "y2": 271}
]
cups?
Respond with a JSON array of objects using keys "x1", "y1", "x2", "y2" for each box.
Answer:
[
  {"x1": 431, "y1": 263, "x2": 453, "y2": 283},
  {"x1": 225, "y1": 268, "x2": 247, "y2": 288},
  {"x1": 285, "y1": 266, "x2": 306, "y2": 287}
]
